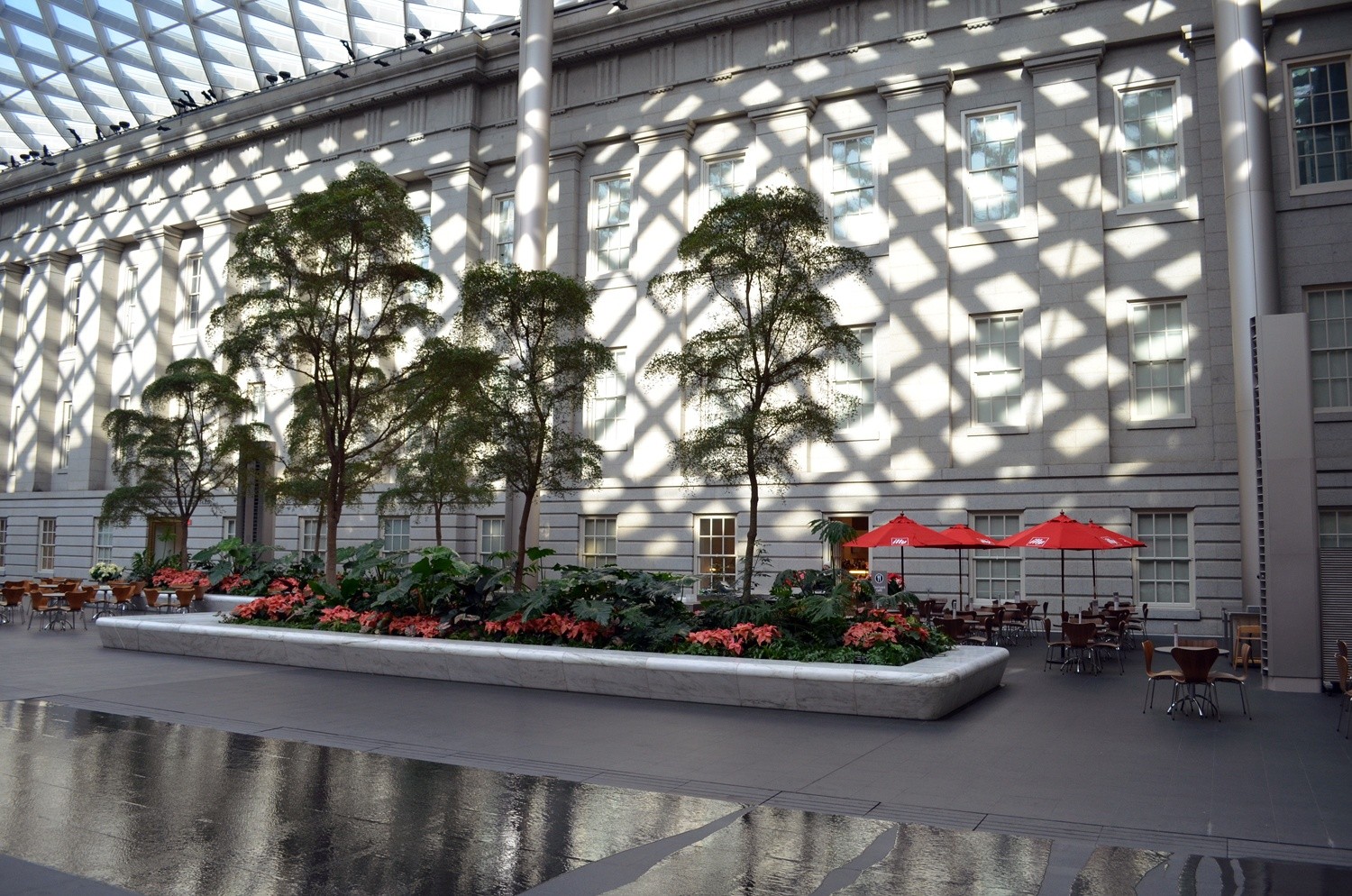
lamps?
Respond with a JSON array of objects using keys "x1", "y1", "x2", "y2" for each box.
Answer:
[{"x1": 0, "y1": 1, "x2": 627, "y2": 176}]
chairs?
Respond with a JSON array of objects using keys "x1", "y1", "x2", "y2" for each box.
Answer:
[
  {"x1": 0, "y1": 576, "x2": 206, "y2": 633},
  {"x1": 916, "y1": 591, "x2": 1351, "y2": 743}
]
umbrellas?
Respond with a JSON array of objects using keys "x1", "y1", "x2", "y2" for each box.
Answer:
[
  {"x1": 843, "y1": 512, "x2": 1011, "y2": 613},
  {"x1": 998, "y1": 511, "x2": 1148, "y2": 658}
]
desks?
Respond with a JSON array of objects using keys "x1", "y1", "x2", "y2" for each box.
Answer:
[
  {"x1": 93, "y1": 588, "x2": 117, "y2": 618},
  {"x1": 52, "y1": 579, "x2": 66, "y2": 583},
  {"x1": 1099, "y1": 605, "x2": 1136, "y2": 609},
  {"x1": 109, "y1": 582, "x2": 132, "y2": 585},
  {"x1": 901, "y1": 602, "x2": 946, "y2": 608},
  {"x1": 1075, "y1": 611, "x2": 1139, "y2": 617},
  {"x1": 40, "y1": 592, "x2": 75, "y2": 632},
  {"x1": 1053, "y1": 622, "x2": 1107, "y2": 677},
  {"x1": 1006, "y1": 602, "x2": 1041, "y2": 638},
  {"x1": 1226, "y1": 611, "x2": 1261, "y2": 664},
  {"x1": 37, "y1": 584, "x2": 59, "y2": 592},
  {"x1": 971, "y1": 611, "x2": 995, "y2": 636},
  {"x1": 931, "y1": 617, "x2": 979, "y2": 644},
  {"x1": 170, "y1": 584, "x2": 196, "y2": 589},
  {"x1": 974, "y1": 607, "x2": 1021, "y2": 645},
  {"x1": 158, "y1": 590, "x2": 179, "y2": 614},
  {"x1": 1155, "y1": 646, "x2": 1230, "y2": 722}
]
